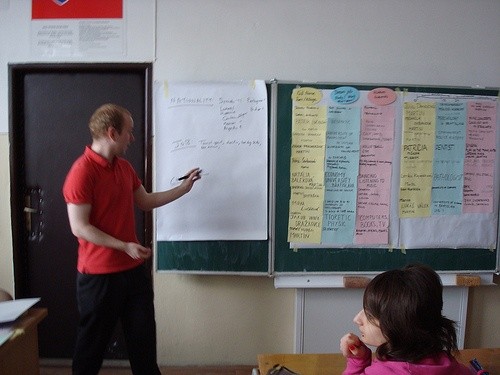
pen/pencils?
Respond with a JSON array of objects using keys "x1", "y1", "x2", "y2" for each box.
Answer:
[{"x1": 176, "y1": 168, "x2": 202, "y2": 181}]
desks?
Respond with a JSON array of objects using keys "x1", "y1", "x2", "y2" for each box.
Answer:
[
  {"x1": 0, "y1": 305, "x2": 49, "y2": 375},
  {"x1": 255, "y1": 348, "x2": 499, "y2": 375}
]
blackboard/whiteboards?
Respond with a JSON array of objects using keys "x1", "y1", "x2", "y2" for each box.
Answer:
[{"x1": 153, "y1": 81, "x2": 500, "y2": 275}]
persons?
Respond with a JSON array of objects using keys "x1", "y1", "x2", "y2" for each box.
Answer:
[
  {"x1": 340, "y1": 265, "x2": 474, "y2": 375},
  {"x1": 63, "y1": 103, "x2": 202, "y2": 375}
]
